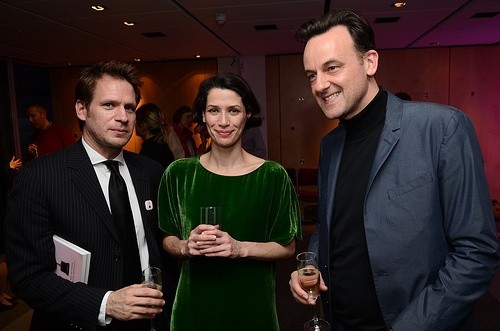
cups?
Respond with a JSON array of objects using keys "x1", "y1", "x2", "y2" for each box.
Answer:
[{"x1": 199, "y1": 207, "x2": 217, "y2": 226}]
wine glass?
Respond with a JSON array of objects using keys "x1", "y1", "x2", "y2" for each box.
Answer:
[
  {"x1": 143, "y1": 268, "x2": 163, "y2": 331},
  {"x1": 296, "y1": 252, "x2": 330, "y2": 331}
]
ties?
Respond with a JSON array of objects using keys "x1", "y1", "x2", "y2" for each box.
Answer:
[{"x1": 103, "y1": 159, "x2": 142, "y2": 284}]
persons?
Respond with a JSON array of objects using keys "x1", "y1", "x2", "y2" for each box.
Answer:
[
  {"x1": 158, "y1": 74, "x2": 303, "y2": 331},
  {"x1": 9, "y1": 60, "x2": 165, "y2": 331},
  {"x1": 1, "y1": 102, "x2": 76, "y2": 310},
  {"x1": 132, "y1": 103, "x2": 266, "y2": 167},
  {"x1": 289, "y1": 9, "x2": 496, "y2": 331}
]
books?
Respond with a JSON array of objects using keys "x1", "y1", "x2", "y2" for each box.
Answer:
[{"x1": 52, "y1": 234, "x2": 91, "y2": 284}]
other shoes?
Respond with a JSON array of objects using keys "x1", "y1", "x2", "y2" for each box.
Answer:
[{"x1": 0, "y1": 296, "x2": 18, "y2": 310}]
